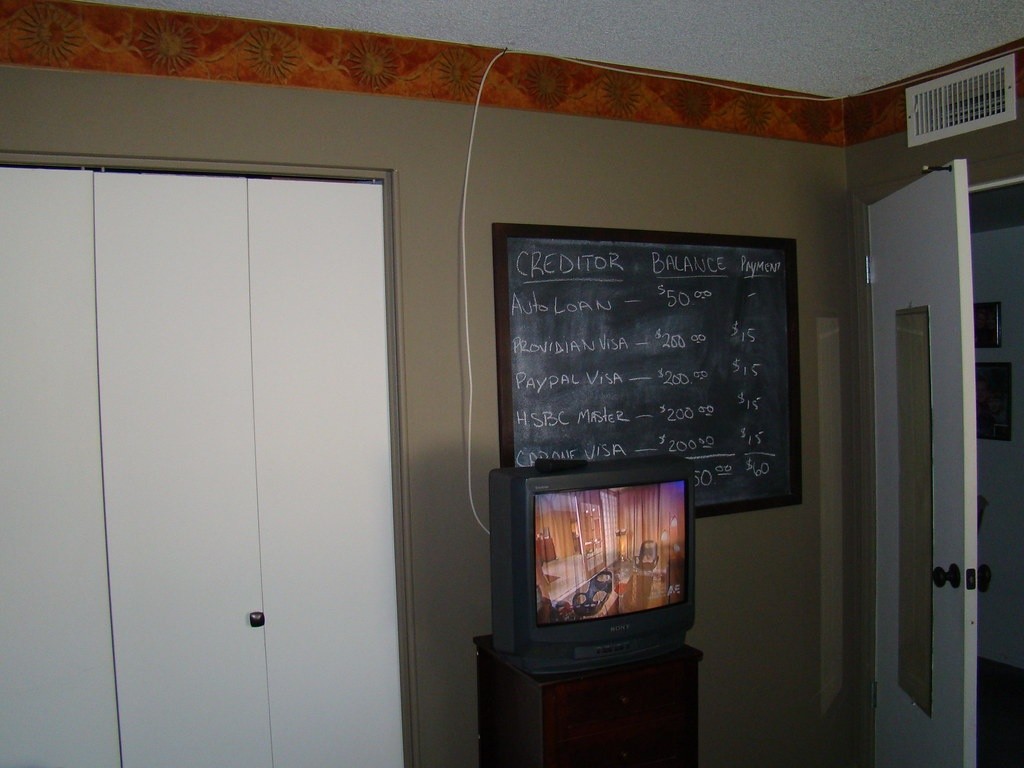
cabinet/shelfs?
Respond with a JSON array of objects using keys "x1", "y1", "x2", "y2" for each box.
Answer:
[{"x1": 473, "y1": 633, "x2": 704, "y2": 768}]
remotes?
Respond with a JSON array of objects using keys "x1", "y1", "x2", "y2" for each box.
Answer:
[{"x1": 534, "y1": 458, "x2": 587, "y2": 475}]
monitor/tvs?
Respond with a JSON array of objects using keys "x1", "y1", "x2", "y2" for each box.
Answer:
[{"x1": 488, "y1": 452, "x2": 697, "y2": 678}]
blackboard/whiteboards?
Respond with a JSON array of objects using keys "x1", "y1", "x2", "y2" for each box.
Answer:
[{"x1": 490, "y1": 220, "x2": 805, "y2": 522}]
